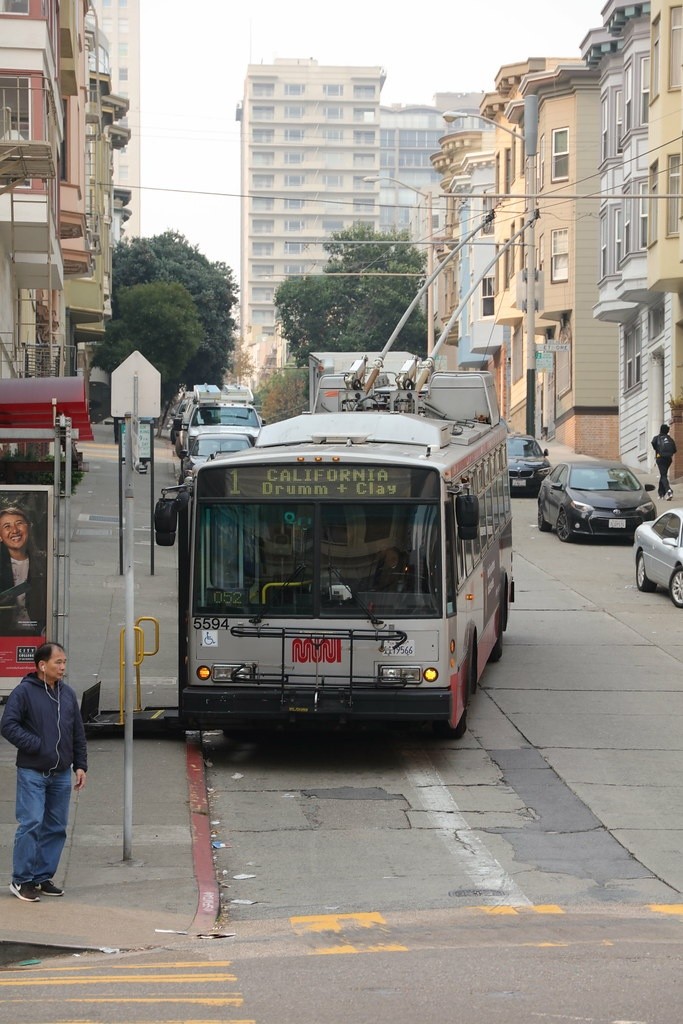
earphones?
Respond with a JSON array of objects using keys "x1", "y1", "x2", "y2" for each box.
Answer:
[{"x1": 42, "y1": 666, "x2": 45, "y2": 672}]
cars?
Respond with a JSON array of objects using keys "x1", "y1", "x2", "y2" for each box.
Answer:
[
  {"x1": 178, "y1": 433, "x2": 257, "y2": 487},
  {"x1": 506, "y1": 436, "x2": 551, "y2": 489},
  {"x1": 633, "y1": 508, "x2": 683, "y2": 608},
  {"x1": 169, "y1": 383, "x2": 268, "y2": 455},
  {"x1": 536, "y1": 459, "x2": 658, "y2": 542}
]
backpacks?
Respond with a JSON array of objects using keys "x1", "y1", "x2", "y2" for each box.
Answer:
[{"x1": 656, "y1": 435, "x2": 677, "y2": 457}]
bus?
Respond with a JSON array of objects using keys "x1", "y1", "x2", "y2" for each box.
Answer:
[{"x1": 152, "y1": 368, "x2": 515, "y2": 739}]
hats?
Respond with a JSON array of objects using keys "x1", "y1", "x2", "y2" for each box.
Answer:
[{"x1": 661, "y1": 425, "x2": 670, "y2": 433}]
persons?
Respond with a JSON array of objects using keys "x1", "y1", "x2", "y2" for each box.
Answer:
[
  {"x1": 651, "y1": 424, "x2": 677, "y2": 501},
  {"x1": 0, "y1": 507, "x2": 47, "y2": 635},
  {"x1": 0, "y1": 641, "x2": 88, "y2": 902}
]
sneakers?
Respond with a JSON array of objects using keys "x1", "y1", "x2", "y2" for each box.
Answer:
[
  {"x1": 38, "y1": 879, "x2": 64, "y2": 896},
  {"x1": 9, "y1": 881, "x2": 40, "y2": 901}
]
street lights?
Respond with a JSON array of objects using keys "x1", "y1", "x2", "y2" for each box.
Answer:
[{"x1": 361, "y1": 173, "x2": 437, "y2": 359}]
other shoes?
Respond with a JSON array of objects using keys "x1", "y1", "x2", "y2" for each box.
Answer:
[
  {"x1": 658, "y1": 495, "x2": 663, "y2": 499},
  {"x1": 666, "y1": 490, "x2": 673, "y2": 501}
]
